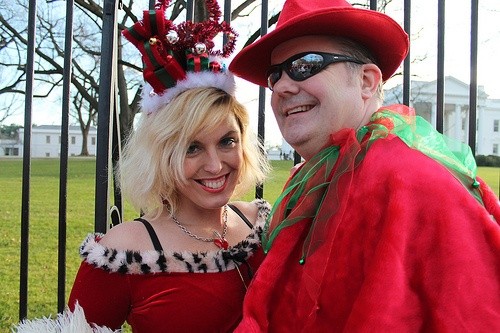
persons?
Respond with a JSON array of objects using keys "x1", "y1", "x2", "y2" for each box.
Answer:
[
  {"x1": 7, "y1": 0, "x2": 271, "y2": 333},
  {"x1": 227, "y1": 0, "x2": 500, "y2": 333}
]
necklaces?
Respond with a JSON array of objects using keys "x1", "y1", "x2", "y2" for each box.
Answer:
[{"x1": 165, "y1": 202, "x2": 229, "y2": 250}]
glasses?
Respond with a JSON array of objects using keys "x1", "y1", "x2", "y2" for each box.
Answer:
[{"x1": 265, "y1": 51, "x2": 367, "y2": 90}]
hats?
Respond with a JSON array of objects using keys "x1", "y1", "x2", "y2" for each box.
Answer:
[{"x1": 228, "y1": 0, "x2": 411, "y2": 86}]
